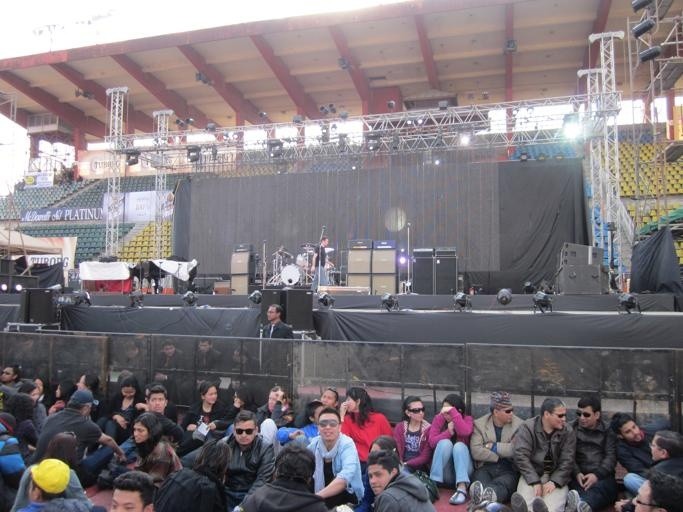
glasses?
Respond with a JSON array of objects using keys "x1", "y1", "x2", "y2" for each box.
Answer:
[
  {"x1": 648, "y1": 443, "x2": 661, "y2": 449},
  {"x1": 235, "y1": 428, "x2": 255, "y2": 434},
  {"x1": 64, "y1": 432, "x2": 80, "y2": 453},
  {"x1": 636, "y1": 493, "x2": 660, "y2": 507},
  {"x1": 407, "y1": 408, "x2": 424, "y2": 413},
  {"x1": 503, "y1": 408, "x2": 513, "y2": 413},
  {"x1": 0, "y1": 371, "x2": 10, "y2": 375},
  {"x1": 552, "y1": 412, "x2": 566, "y2": 417},
  {"x1": 576, "y1": 412, "x2": 590, "y2": 417},
  {"x1": 317, "y1": 419, "x2": 339, "y2": 427}
]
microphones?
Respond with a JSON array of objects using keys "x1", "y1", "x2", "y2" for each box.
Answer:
[
  {"x1": 262, "y1": 238, "x2": 269, "y2": 245},
  {"x1": 406, "y1": 221, "x2": 414, "y2": 229},
  {"x1": 321, "y1": 223, "x2": 328, "y2": 233}
]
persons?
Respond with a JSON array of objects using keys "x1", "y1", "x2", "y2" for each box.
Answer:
[
  {"x1": 311, "y1": 236, "x2": 335, "y2": 292},
  {"x1": 1, "y1": 334, "x2": 683, "y2": 512},
  {"x1": 259, "y1": 302, "x2": 293, "y2": 405}
]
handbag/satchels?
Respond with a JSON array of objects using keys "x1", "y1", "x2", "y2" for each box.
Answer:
[{"x1": 413, "y1": 469, "x2": 440, "y2": 504}]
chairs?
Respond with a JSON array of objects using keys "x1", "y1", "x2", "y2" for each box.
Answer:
[
  {"x1": 584, "y1": 142, "x2": 683, "y2": 278},
  {"x1": 0, "y1": 173, "x2": 174, "y2": 263}
]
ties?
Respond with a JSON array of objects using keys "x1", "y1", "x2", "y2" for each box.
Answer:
[{"x1": 269, "y1": 324, "x2": 274, "y2": 338}]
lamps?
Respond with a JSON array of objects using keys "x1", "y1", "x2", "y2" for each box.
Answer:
[
  {"x1": 258, "y1": 111, "x2": 268, "y2": 119},
  {"x1": 318, "y1": 104, "x2": 336, "y2": 118},
  {"x1": 268, "y1": 139, "x2": 283, "y2": 157},
  {"x1": 630, "y1": 0, "x2": 662, "y2": 62},
  {"x1": 367, "y1": 131, "x2": 380, "y2": 151},
  {"x1": 187, "y1": 145, "x2": 201, "y2": 163},
  {"x1": 126, "y1": 149, "x2": 139, "y2": 166},
  {"x1": 388, "y1": 100, "x2": 395, "y2": 109},
  {"x1": 175, "y1": 117, "x2": 195, "y2": 130}
]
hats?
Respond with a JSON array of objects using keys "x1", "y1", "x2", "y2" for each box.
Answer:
[
  {"x1": 0, "y1": 412, "x2": 16, "y2": 432},
  {"x1": 490, "y1": 391, "x2": 511, "y2": 410},
  {"x1": 30, "y1": 458, "x2": 70, "y2": 494},
  {"x1": 74, "y1": 390, "x2": 99, "y2": 406}
]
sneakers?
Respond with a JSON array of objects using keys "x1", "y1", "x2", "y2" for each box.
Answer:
[
  {"x1": 564, "y1": 489, "x2": 580, "y2": 512},
  {"x1": 483, "y1": 487, "x2": 497, "y2": 502},
  {"x1": 466, "y1": 480, "x2": 483, "y2": 511},
  {"x1": 532, "y1": 498, "x2": 548, "y2": 512},
  {"x1": 510, "y1": 492, "x2": 527, "y2": 512},
  {"x1": 448, "y1": 490, "x2": 467, "y2": 505},
  {"x1": 576, "y1": 501, "x2": 592, "y2": 512}
]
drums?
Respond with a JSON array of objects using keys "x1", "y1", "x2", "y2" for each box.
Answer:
[{"x1": 280, "y1": 264, "x2": 299, "y2": 285}]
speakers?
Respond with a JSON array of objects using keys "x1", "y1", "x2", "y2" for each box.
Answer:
[
  {"x1": 230, "y1": 252, "x2": 256, "y2": 275},
  {"x1": 411, "y1": 257, "x2": 435, "y2": 296},
  {"x1": 347, "y1": 249, "x2": 372, "y2": 275},
  {"x1": 372, "y1": 249, "x2": 397, "y2": 274},
  {"x1": 0, "y1": 259, "x2": 13, "y2": 275},
  {"x1": 435, "y1": 256, "x2": 457, "y2": 296},
  {"x1": 0, "y1": 275, "x2": 12, "y2": 293},
  {"x1": 260, "y1": 288, "x2": 284, "y2": 329},
  {"x1": 230, "y1": 274, "x2": 255, "y2": 295},
  {"x1": 20, "y1": 288, "x2": 27, "y2": 323},
  {"x1": 371, "y1": 274, "x2": 399, "y2": 295},
  {"x1": 283, "y1": 290, "x2": 315, "y2": 331},
  {"x1": 28, "y1": 287, "x2": 54, "y2": 324},
  {"x1": 346, "y1": 274, "x2": 372, "y2": 295},
  {"x1": 12, "y1": 275, "x2": 40, "y2": 293}
]
